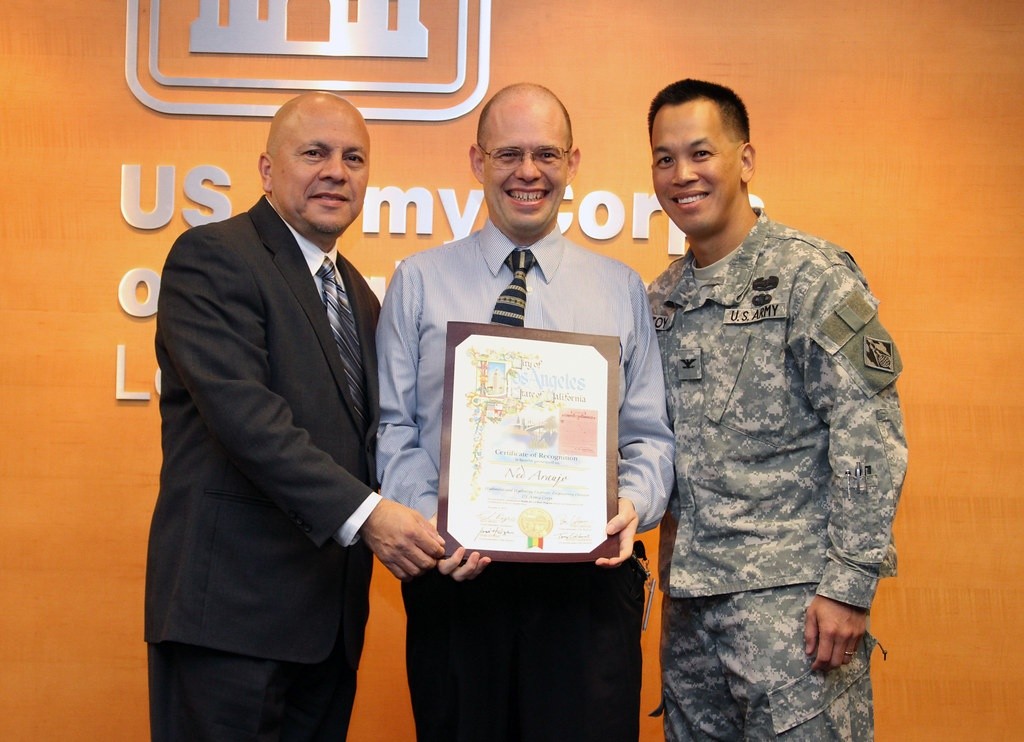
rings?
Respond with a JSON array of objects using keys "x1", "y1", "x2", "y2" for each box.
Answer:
[{"x1": 844, "y1": 652, "x2": 853, "y2": 655}]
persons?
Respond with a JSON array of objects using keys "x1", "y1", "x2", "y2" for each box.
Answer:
[
  {"x1": 140, "y1": 89, "x2": 448, "y2": 741},
  {"x1": 375, "y1": 79, "x2": 677, "y2": 742},
  {"x1": 643, "y1": 77, "x2": 912, "y2": 742}
]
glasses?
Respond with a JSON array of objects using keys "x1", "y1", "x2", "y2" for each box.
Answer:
[{"x1": 479, "y1": 143, "x2": 574, "y2": 169}]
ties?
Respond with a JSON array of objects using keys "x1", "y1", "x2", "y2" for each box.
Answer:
[
  {"x1": 318, "y1": 255, "x2": 366, "y2": 427},
  {"x1": 491, "y1": 248, "x2": 535, "y2": 326}
]
